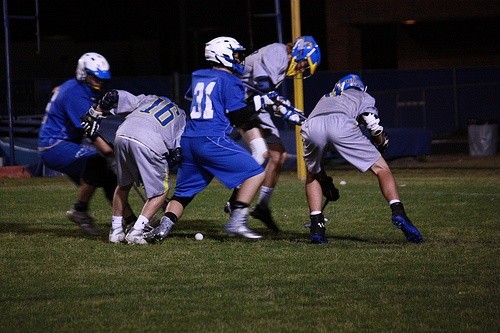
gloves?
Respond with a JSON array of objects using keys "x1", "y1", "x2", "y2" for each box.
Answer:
[
  {"x1": 80, "y1": 106, "x2": 105, "y2": 140},
  {"x1": 372, "y1": 129, "x2": 391, "y2": 153},
  {"x1": 253, "y1": 91, "x2": 279, "y2": 112},
  {"x1": 273, "y1": 94, "x2": 305, "y2": 125},
  {"x1": 322, "y1": 175, "x2": 339, "y2": 202}
]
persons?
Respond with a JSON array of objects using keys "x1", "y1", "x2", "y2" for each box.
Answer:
[
  {"x1": 38, "y1": 37, "x2": 322, "y2": 245},
  {"x1": 301, "y1": 74, "x2": 420, "y2": 244}
]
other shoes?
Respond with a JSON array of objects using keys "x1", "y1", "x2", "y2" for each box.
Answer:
[
  {"x1": 144, "y1": 225, "x2": 169, "y2": 243},
  {"x1": 109, "y1": 230, "x2": 127, "y2": 243},
  {"x1": 126, "y1": 226, "x2": 148, "y2": 244},
  {"x1": 66, "y1": 209, "x2": 99, "y2": 235},
  {"x1": 124, "y1": 220, "x2": 156, "y2": 238},
  {"x1": 251, "y1": 205, "x2": 281, "y2": 233},
  {"x1": 223, "y1": 201, "x2": 237, "y2": 214},
  {"x1": 224, "y1": 222, "x2": 262, "y2": 241}
]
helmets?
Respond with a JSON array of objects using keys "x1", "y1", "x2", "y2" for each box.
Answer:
[
  {"x1": 205, "y1": 36, "x2": 246, "y2": 75},
  {"x1": 287, "y1": 35, "x2": 320, "y2": 80},
  {"x1": 333, "y1": 74, "x2": 365, "y2": 92},
  {"x1": 76, "y1": 52, "x2": 111, "y2": 80}
]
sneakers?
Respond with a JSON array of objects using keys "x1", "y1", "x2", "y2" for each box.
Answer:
[
  {"x1": 310, "y1": 229, "x2": 327, "y2": 244},
  {"x1": 391, "y1": 210, "x2": 424, "y2": 242}
]
